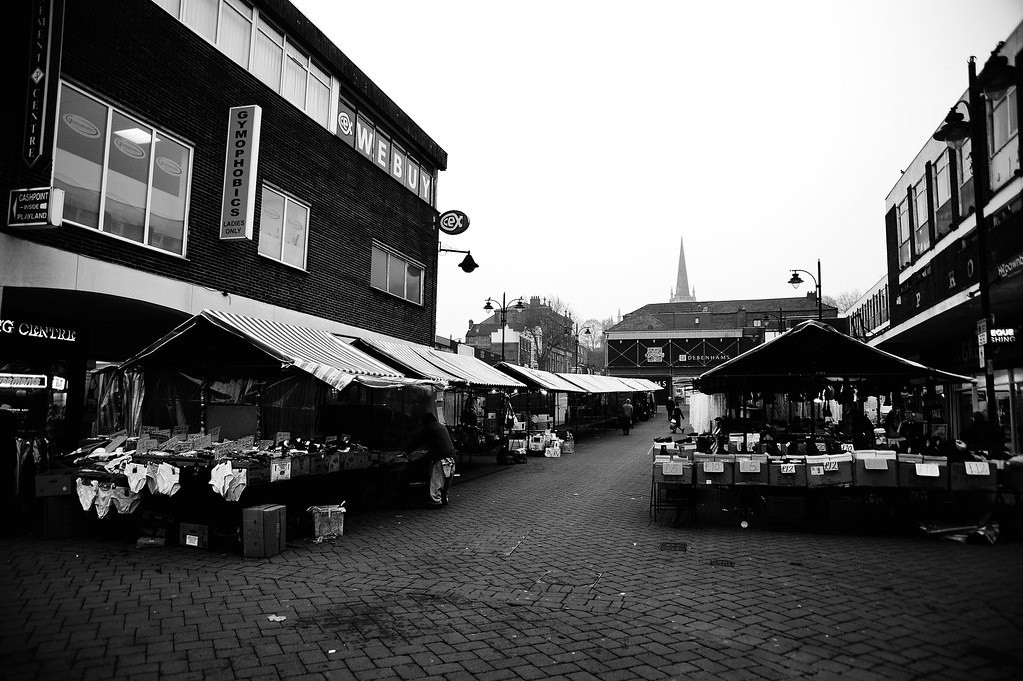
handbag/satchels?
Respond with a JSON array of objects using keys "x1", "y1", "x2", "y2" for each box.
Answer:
[{"x1": 670, "y1": 419, "x2": 676, "y2": 429}]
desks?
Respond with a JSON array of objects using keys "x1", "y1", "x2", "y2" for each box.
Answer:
[{"x1": 511, "y1": 428, "x2": 545, "y2": 438}]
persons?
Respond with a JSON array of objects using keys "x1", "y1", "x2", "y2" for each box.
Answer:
[
  {"x1": 666, "y1": 396, "x2": 683, "y2": 433},
  {"x1": 404, "y1": 412, "x2": 456, "y2": 505},
  {"x1": 964, "y1": 410, "x2": 994, "y2": 448},
  {"x1": 622, "y1": 398, "x2": 634, "y2": 435}
]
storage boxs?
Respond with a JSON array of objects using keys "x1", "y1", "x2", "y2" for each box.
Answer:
[
  {"x1": 653, "y1": 440, "x2": 1023, "y2": 492},
  {"x1": 242, "y1": 504, "x2": 286, "y2": 559},
  {"x1": 34, "y1": 471, "x2": 72, "y2": 498},
  {"x1": 767, "y1": 495, "x2": 804, "y2": 519},
  {"x1": 509, "y1": 413, "x2": 574, "y2": 458},
  {"x1": 313, "y1": 504, "x2": 346, "y2": 538},
  {"x1": 178, "y1": 523, "x2": 209, "y2": 549}
]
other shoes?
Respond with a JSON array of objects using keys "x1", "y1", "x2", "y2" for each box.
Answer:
[
  {"x1": 682, "y1": 428, "x2": 684, "y2": 434},
  {"x1": 671, "y1": 431, "x2": 676, "y2": 433}
]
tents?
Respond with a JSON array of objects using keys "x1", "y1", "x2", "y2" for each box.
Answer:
[
  {"x1": 117, "y1": 308, "x2": 666, "y2": 447},
  {"x1": 694, "y1": 318, "x2": 979, "y2": 437}
]
